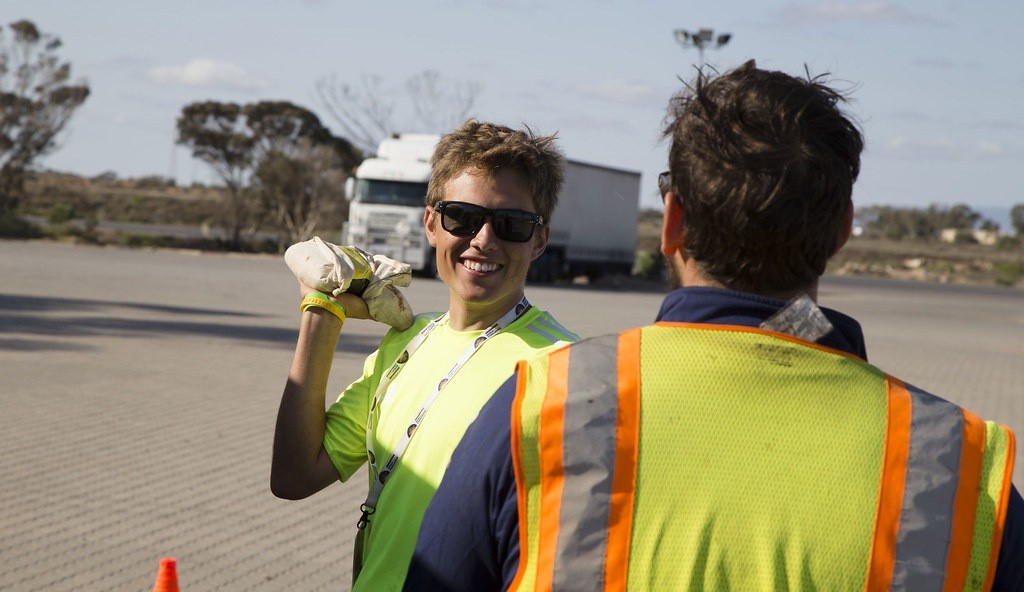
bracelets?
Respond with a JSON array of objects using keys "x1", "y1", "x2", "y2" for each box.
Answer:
[{"x1": 300, "y1": 291, "x2": 346, "y2": 322}]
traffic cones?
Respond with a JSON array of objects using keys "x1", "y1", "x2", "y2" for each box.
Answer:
[{"x1": 152, "y1": 558, "x2": 180, "y2": 592}]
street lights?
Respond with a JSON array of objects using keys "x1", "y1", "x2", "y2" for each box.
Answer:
[{"x1": 673, "y1": 27, "x2": 732, "y2": 89}]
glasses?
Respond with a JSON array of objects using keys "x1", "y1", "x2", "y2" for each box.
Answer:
[
  {"x1": 658, "y1": 171, "x2": 673, "y2": 204},
  {"x1": 434, "y1": 200, "x2": 544, "y2": 243}
]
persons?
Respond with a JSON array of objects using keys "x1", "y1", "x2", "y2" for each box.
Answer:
[
  {"x1": 404, "y1": 59, "x2": 1024, "y2": 592},
  {"x1": 271, "y1": 117, "x2": 583, "y2": 592}
]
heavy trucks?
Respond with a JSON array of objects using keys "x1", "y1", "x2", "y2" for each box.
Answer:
[{"x1": 343, "y1": 133, "x2": 641, "y2": 284}]
